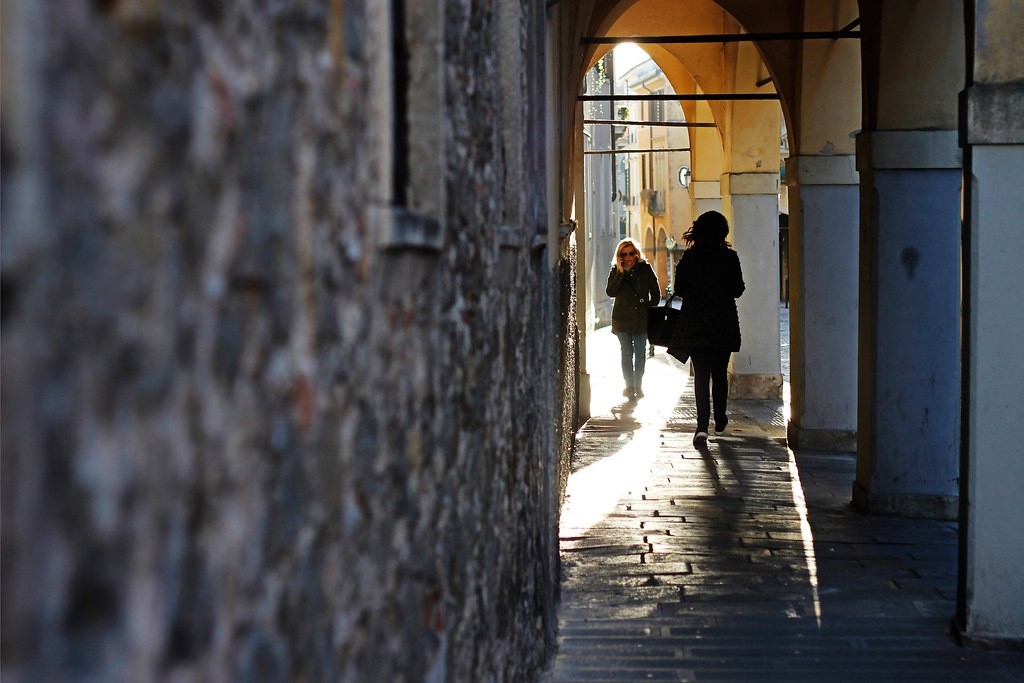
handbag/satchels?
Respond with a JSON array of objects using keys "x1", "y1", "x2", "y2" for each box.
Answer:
[{"x1": 646, "y1": 293, "x2": 681, "y2": 346}]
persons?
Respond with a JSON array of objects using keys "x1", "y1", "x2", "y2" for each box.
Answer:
[
  {"x1": 673, "y1": 209, "x2": 746, "y2": 444},
  {"x1": 606, "y1": 238, "x2": 663, "y2": 399}
]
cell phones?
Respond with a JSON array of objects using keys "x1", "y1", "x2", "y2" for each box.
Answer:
[{"x1": 619, "y1": 255, "x2": 624, "y2": 267}]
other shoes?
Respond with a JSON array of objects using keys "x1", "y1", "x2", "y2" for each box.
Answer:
[
  {"x1": 713, "y1": 414, "x2": 728, "y2": 436},
  {"x1": 693, "y1": 427, "x2": 708, "y2": 443},
  {"x1": 623, "y1": 386, "x2": 644, "y2": 400}
]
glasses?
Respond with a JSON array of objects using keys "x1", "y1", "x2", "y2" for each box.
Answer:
[{"x1": 620, "y1": 252, "x2": 635, "y2": 258}]
